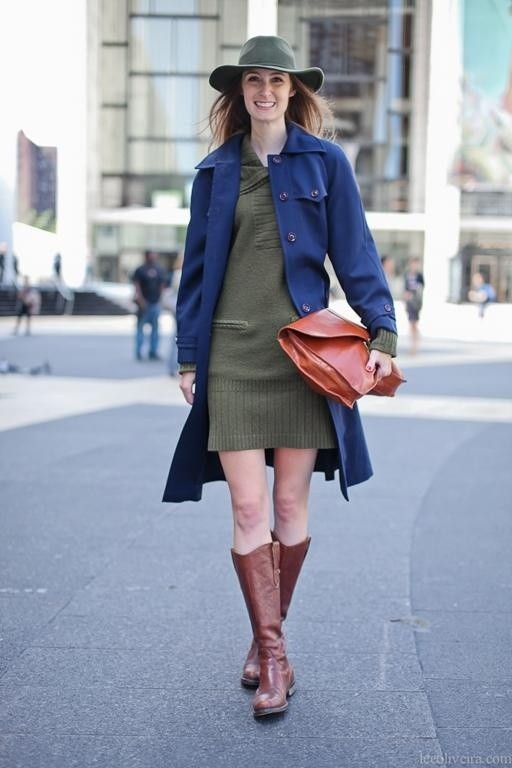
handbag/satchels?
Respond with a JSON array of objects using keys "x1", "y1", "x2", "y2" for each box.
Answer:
[{"x1": 277, "y1": 308, "x2": 407, "y2": 410}]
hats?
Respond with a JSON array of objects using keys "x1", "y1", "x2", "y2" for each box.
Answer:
[{"x1": 209, "y1": 35, "x2": 325, "y2": 96}]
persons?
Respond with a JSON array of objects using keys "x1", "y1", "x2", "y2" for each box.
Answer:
[
  {"x1": 130, "y1": 249, "x2": 165, "y2": 361},
  {"x1": 12, "y1": 275, "x2": 34, "y2": 336},
  {"x1": 381, "y1": 254, "x2": 423, "y2": 356},
  {"x1": 161, "y1": 36, "x2": 407, "y2": 716}
]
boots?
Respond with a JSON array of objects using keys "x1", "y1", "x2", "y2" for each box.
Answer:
[{"x1": 231, "y1": 530, "x2": 311, "y2": 716}]
